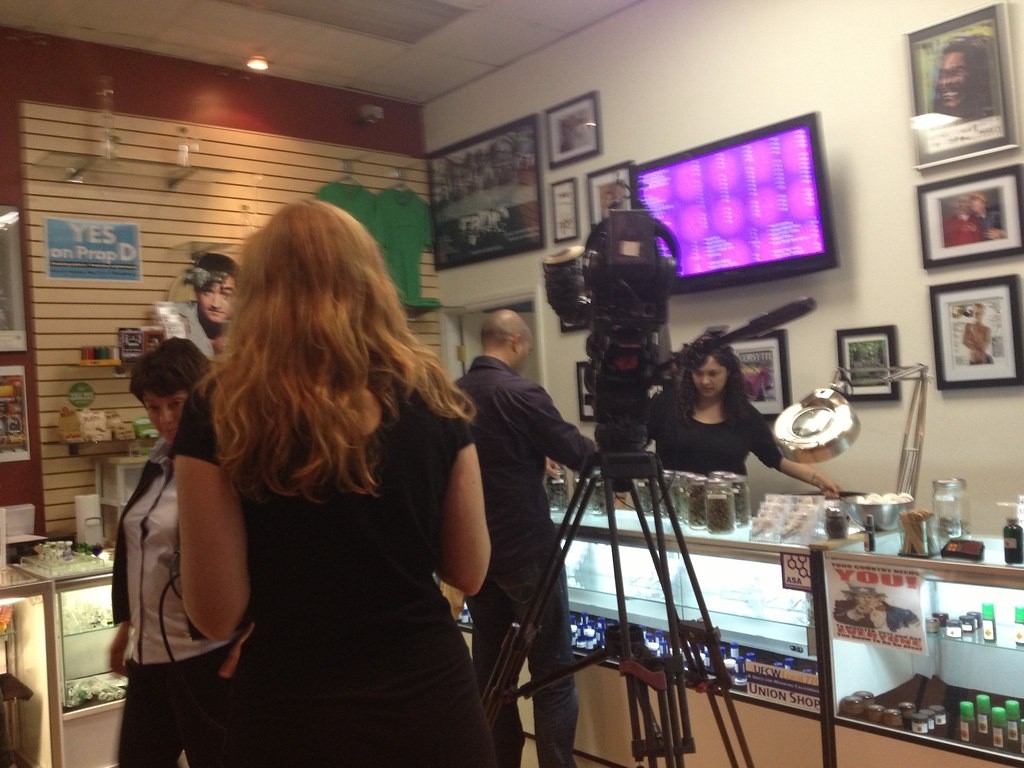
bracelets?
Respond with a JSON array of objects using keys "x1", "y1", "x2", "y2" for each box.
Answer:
[
  {"x1": 810, "y1": 471, "x2": 818, "y2": 485},
  {"x1": 615, "y1": 496, "x2": 627, "y2": 501}
]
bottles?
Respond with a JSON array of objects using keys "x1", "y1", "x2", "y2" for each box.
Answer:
[
  {"x1": 959, "y1": 695, "x2": 1024, "y2": 754},
  {"x1": 458, "y1": 600, "x2": 819, "y2": 695},
  {"x1": 546, "y1": 467, "x2": 753, "y2": 535},
  {"x1": 825, "y1": 507, "x2": 846, "y2": 539},
  {"x1": 1014, "y1": 605, "x2": 1024, "y2": 646},
  {"x1": 1003, "y1": 517, "x2": 1024, "y2": 565},
  {"x1": 934, "y1": 477, "x2": 972, "y2": 546},
  {"x1": 982, "y1": 603, "x2": 997, "y2": 644},
  {"x1": 864, "y1": 514, "x2": 875, "y2": 552}
]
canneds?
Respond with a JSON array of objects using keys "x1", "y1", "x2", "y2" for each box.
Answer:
[
  {"x1": 825, "y1": 507, "x2": 849, "y2": 539},
  {"x1": 584, "y1": 470, "x2": 608, "y2": 516},
  {"x1": 545, "y1": 468, "x2": 570, "y2": 513},
  {"x1": 635, "y1": 470, "x2": 750, "y2": 534}
]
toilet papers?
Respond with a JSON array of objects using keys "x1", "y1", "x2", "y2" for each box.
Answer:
[{"x1": 74, "y1": 493, "x2": 104, "y2": 549}]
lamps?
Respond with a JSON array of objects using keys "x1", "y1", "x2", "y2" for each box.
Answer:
[{"x1": 245, "y1": 55, "x2": 270, "y2": 71}]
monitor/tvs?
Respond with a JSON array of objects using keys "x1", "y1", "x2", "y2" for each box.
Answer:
[{"x1": 627, "y1": 113, "x2": 840, "y2": 295}]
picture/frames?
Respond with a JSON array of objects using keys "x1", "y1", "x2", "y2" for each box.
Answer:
[
  {"x1": 916, "y1": 163, "x2": 1024, "y2": 269},
  {"x1": 424, "y1": 112, "x2": 544, "y2": 274},
  {"x1": 903, "y1": 0, "x2": 1021, "y2": 170},
  {"x1": 723, "y1": 329, "x2": 792, "y2": 419},
  {"x1": 550, "y1": 176, "x2": 582, "y2": 242},
  {"x1": 928, "y1": 273, "x2": 1024, "y2": 391},
  {"x1": 577, "y1": 361, "x2": 597, "y2": 421},
  {"x1": 544, "y1": 89, "x2": 606, "y2": 166},
  {"x1": 587, "y1": 159, "x2": 637, "y2": 235},
  {"x1": 834, "y1": 324, "x2": 902, "y2": 403}
]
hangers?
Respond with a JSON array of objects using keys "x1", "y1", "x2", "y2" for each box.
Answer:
[
  {"x1": 335, "y1": 161, "x2": 361, "y2": 186},
  {"x1": 389, "y1": 166, "x2": 410, "y2": 189}
]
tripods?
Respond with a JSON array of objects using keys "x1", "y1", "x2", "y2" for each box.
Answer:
[{"x1": 477, "y1": 297, "x2": 816, "y2": 768}]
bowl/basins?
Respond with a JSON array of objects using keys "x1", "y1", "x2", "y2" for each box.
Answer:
[{"x1": 843, "y1": 499, "x2": 915, "y2": 532}]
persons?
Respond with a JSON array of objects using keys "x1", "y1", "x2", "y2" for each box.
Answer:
[
  {"x1": 168, "y1": 200, "x2": 502, "y2": 768},
  {"x1": 448, "y1": 310, "x2": 601, "y2": 768},
  {"x1": 110, "y1": 338, "x2": 255, "y2": 768},
  {"x1": 835, "y1": 585, "x2": 920, "y2": 636},
  {"x1": 963, "y1": 304, "x2": 992, "y2": 365},
  {"x1": 606, "y1": 192, "x2": 617, "y2": 219},
  {"x1": 740, "y1": 361, "x2": 773, "y2": 402},
  {"x1": 608, "y1": 337, "x2": 845, "y2": 509},
  {"x1": 173, "y1": 253, "x2": 240, "y2": 359},
  {"x1": 936, "y1": 40, "x2": 993, "y2": 121},
  {"x1": 430, "y1": 132, "x2": 537, "y2": 257},
  {"x1": 943, "y1": 192, "x2": 1006, "y2": 247}
]
